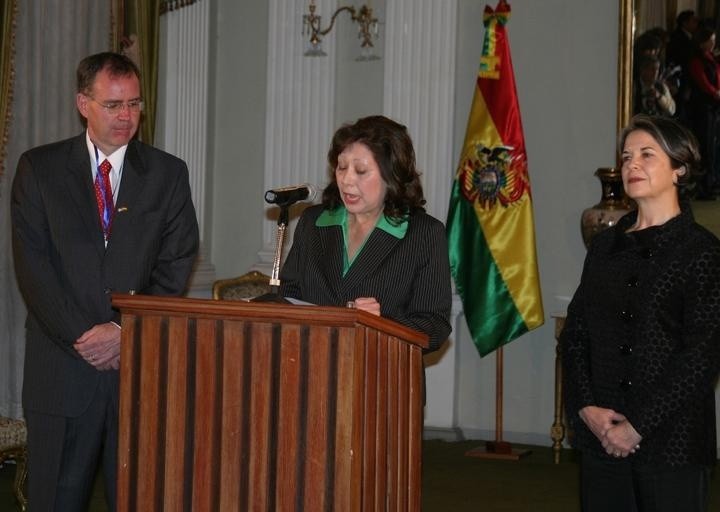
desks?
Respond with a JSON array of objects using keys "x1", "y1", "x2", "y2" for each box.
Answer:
[{"x1": 550, "y1": 296, "x2": 571, "y2": 465}]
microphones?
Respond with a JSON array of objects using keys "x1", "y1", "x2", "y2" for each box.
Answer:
[{"x1": 264, "y1": 182, "x2": 318, "y2": 204}]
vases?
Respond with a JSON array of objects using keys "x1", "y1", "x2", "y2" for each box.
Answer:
[{"x1": 581, "y1": 166, "x2": 637, "y2": 251}]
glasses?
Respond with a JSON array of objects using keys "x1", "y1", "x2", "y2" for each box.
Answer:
[{"x1": 82, "y1": 93, "x2": 146, "y2": 114}]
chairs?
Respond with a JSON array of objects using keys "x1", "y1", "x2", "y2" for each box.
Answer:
[
  {"x1": 0, "y1": 415, "x2": 28, "y2": 512},
  {"x1": 213, "y1": 271, "x2": 270, "y2": 301}
]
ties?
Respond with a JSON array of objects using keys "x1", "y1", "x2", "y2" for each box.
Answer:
[{"x1": 93, "y1": 158, "x2": 115, "y2": 243}]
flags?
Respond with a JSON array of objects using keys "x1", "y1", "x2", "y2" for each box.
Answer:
[{"x1": 446, "y1": 0, "x2": 546, "y2": 358}]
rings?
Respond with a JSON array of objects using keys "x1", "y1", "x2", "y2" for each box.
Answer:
[
  {"x1": 90, "y1": 355, "x2": 96, "y2": 361},
  {"x1": 613, "y1": 452, "x2": 620, "y2": 458}
]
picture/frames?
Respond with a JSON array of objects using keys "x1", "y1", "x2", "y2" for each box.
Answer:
[{"x1": 617, "y1": 0, "x2": 720, "y2": 200}]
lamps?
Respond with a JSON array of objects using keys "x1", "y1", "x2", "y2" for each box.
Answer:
[{"x1": 302, "y1": 0, "x2": 380, "y2": 62}]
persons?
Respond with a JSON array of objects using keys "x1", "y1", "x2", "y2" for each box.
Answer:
[
  {"x1": 558, "y1": 112, "x2": 720, "y2": 512},
  {"x1": 632, "y1": 9, "x2": 720, "y2": 187},
  {"x1": 279, "y1": 115, "x2": 452, "y2": 407},
  {"x1": 11, "y1": 51, "x2": 199, "y2": 512}
]
